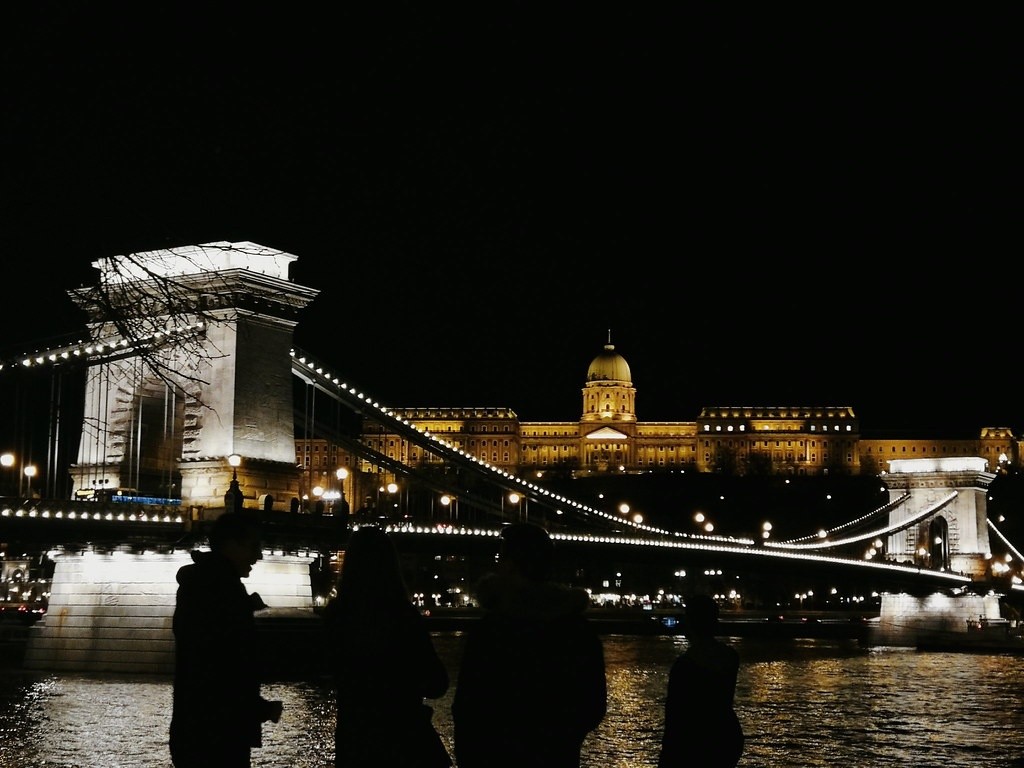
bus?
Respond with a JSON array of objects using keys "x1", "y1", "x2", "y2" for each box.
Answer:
[{"x1": 73, "y1": 486, "x2": 139, "y2": 503}]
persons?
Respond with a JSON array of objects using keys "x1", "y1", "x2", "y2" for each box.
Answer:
[
  {"x1": 447, "y1": 522, "x2": 607, "y2": 768},
  {"x1": 659, "y1": 595, "x2": 746, "y2": 768},
  {"x1": 299, "y1": 529, "x2": 458, "y2": 768},
  {"x1": 166, "y1": 510, "x2": 285, "y2": 768}
]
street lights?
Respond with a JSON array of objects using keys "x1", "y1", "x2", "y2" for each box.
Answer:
[
  {"x1": 387, "y1": 482, "x2": 402, "y2": 516},
  {"x1": 221, "y1": 452, "x2": 245, "y2": 513},
  {"x1": 0, "y1": 450, "x2": 17, "y2": 496},
  {"x1": 330, "y1": 467, "x2": 351, "y2": 517},
  {"x1": 440, "y1": 494, "x2": 453, "y2": 521},
  {"x1": 24, "y1": 464, "x2": 38, "y2": 499},
  {"x1": 509, "y1": 493, "x2": 523, "y2": 522}
]
